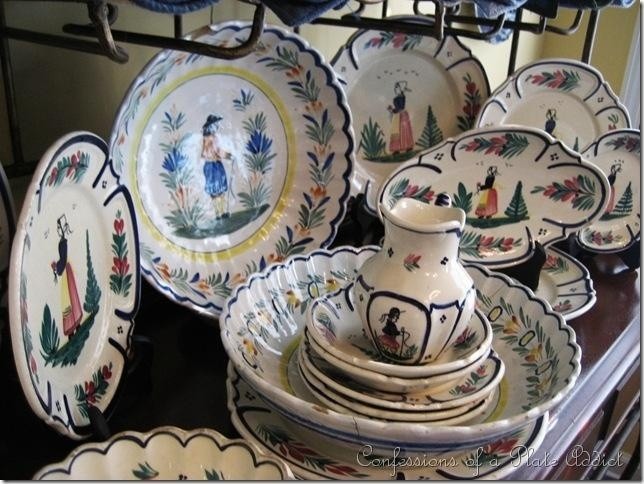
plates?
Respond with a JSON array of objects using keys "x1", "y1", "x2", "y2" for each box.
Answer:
[{"x1": 8, "y1": 16, "x2": 640, "y2": 481}]
